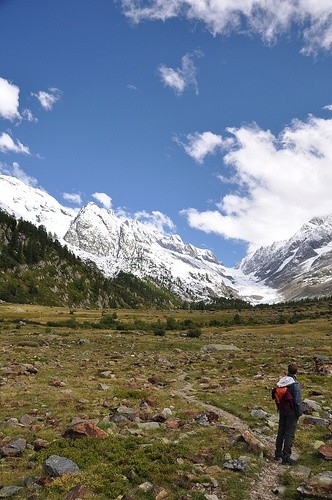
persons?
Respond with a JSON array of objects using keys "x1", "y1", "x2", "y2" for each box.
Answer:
[{"x1": 271, "y1": 363, "x2": 303, "y2": 465}]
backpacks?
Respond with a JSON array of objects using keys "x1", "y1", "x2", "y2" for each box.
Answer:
[{"x1": 274, "y1": 387, "x2": 294, "y2": 413}]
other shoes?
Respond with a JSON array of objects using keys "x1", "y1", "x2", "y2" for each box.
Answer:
[
  {"x1": 283, "y1": 458, "x2": 297, "y2": 465},
  {"x1": 274, "y1": 457, "x2": 283, "y2": 462}
]
